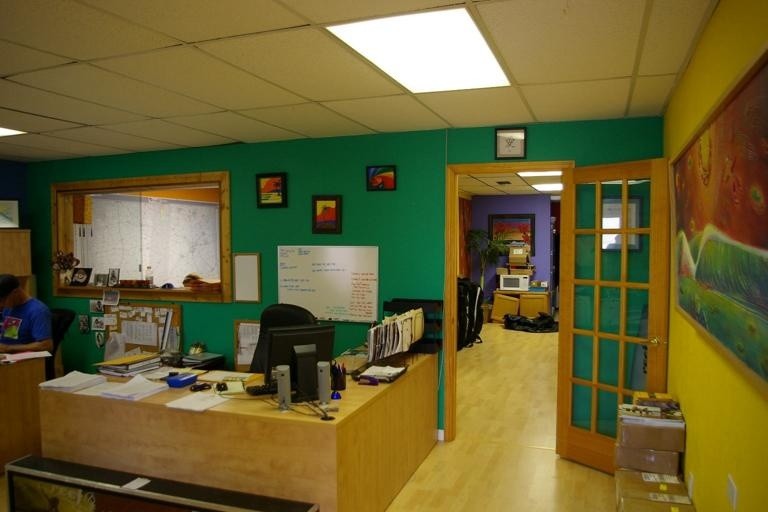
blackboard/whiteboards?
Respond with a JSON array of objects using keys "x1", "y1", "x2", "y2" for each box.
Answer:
[{"x1": 277, "y1": 246, "x2": 379, "y2": 324}]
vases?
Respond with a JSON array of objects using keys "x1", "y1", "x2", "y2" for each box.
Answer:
[{"x1": 63, "y1": 269, "x2": 72, "y2": 286}]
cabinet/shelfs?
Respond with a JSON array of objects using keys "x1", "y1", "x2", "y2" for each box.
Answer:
[
  {"x1": 0, "y1": 357, "x2": 45, "y2": 475},
  {"x1": 0, "y1": 228, "x2": 36, "y2": 298},
  {"x1": 382, "y1": 298, "x2": 442, "y2": 354},
  {"x1": 490, "y1": 288, "x2": 551, "y2": 323}
]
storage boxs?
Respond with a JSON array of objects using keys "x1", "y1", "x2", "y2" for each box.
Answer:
[
  {"x1": 495, "y1": 247, "x2": 536, "y2": 276},
  {"x1": 613, "y1": 392, "x2": 695, "y2": 511}
]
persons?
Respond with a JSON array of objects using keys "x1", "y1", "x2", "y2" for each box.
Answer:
[
  {"x1": 93, "y1": 318, "x2": 104, "y2": 329},
  {"x1": 176, "y1": 269, "x2": 202, "y2": 292},
  {"x1": 95, "y1": 300, "x2": 102, "y2": 312},
  {"x1": 0, "y1": 273, "x2": 53, "y2": 353}
]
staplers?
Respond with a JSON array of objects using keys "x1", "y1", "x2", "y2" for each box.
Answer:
[{"x1": 358, "y1": 375, "x2": 379, "y2": 385}]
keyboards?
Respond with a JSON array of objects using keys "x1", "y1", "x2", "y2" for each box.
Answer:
[{"x1": 245, "y1": 384, "x2": 278, "y2": 396}]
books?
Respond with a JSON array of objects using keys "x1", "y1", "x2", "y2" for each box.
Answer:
[
  {"x1": 181, "y1": 351, "x2": 223, "y2": 369},
  {"x1": 367, "y1": 307, "x2": 425, "y2": 364},
  {"x1": 93, "y1": 353, "x2": 162, "y2": 377},
  {"x1": 631, "y1": 389, "x2": 674, "y2": 406},
  {"x1": 212, "y1": 378, "x2": 246, "y2": 396}
]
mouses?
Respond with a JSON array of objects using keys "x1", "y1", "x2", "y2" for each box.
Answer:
[{"x1": 216, "y1": 382, "x2": 228, "y2": 391}]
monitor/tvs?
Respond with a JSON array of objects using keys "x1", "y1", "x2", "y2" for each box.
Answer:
[{"x1": 264, "y1": 323, "x2": 335, "y2": 403}]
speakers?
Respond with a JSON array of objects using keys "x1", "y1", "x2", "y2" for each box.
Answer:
[
  {"x1": 276, "y1": 365, "x2": 292, "y2": 410},
  {"x1": 316, "y1": 361, "x2": 332, "y2": 405}
]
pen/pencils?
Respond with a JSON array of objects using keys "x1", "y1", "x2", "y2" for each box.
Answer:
[{"x1": 332, "y1": 362, "x2": 346, "y2": 389}]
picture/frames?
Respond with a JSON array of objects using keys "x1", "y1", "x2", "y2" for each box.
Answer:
[
  {"x1": 487, "y1": 213, "x2": 535, "y2": 257},
  {"x1": 365, "y1": 165, "x2": 396, "y2": 191},
  {"x1": 669, "y1": 40, "x2": 767, "y2": 405},
  {"x1": 94, "y1": 274, "x2": 108, "y2": 287},
  {"x1": 494, "y1": 127, "x2": 526, "y2": 160},
  {"x1": 108, "y1": 268, "x2": 120, "y2": 286},
  {"x1": 0, "y1": 198, "x2": 21, "y2": 230},
  {"x1": 311, "y1": 194, "x2": 342, "y2": 234},
  {"x1": 601, "y1": 195, "x2": 642, "y2": 253},
  {"x1": 255, "y1": 172, "x2": 287, "y2": 208}
]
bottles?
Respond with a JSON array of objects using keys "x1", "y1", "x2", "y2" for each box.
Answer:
[{"x1": 144, "y1": 266, "x2": 153, "y2": 290}]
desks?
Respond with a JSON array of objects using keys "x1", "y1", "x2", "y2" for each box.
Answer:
[
  {"x1": 161, "y1": 352, "x2": 225, "y2": 371},
  {"x1": 36, "y1": 346, "x2": 439, "y2": 512}
]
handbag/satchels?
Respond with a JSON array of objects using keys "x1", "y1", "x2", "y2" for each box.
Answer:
[{"x1": 457, "y1": 276, "x2": 484, "y2": 351}]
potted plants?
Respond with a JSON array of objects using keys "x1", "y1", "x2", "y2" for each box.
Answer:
[{"x1": 464, "y1": 229, "x2": 509, "y2": 323}]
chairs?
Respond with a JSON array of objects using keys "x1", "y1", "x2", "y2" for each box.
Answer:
[
  {"x1": 44, "y1": 308, "x2": 76, "y2": 381},
  {"x1": 248, "y1": 303, "x2": 317, "y2": 373}
]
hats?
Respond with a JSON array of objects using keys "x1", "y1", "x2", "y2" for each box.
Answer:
[{"x1": 0, "y1": 274, "x2": 18, "y2": 298}]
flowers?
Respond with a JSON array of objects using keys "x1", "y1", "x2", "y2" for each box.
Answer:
[{"x1": 50, "y1": 250, "x2": 80, "y2": 271}]
